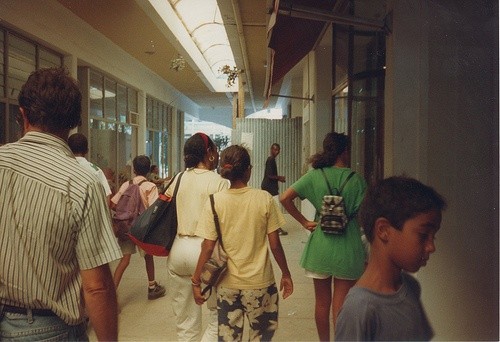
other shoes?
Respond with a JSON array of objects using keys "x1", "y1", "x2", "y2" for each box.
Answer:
[
  {"x1": 277, "y1": 228, "x2": 288, "y2": 235},
  {"x1": 149, "y1": 283, "x2": 167, "y2": 299}
]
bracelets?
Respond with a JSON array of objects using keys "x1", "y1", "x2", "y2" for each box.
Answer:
[{"x1": 191, "y1": 276, "x2": 200, "y2": 286}]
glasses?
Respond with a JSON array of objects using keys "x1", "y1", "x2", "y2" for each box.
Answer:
[{"x1": 16, "y1": 116, "x2": 24, "y2": 127}]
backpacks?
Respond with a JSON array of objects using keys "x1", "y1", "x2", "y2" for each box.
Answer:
[
  {"x1": 317, "y1": 168, "x2": 361, "y2": 235},
  {"x1": 112, "y1": 177, "x2": 148, "y2": 242}
]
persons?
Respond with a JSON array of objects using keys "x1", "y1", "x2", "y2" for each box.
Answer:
[
  {"x1": 150, "y1": 165, "x2": 164, "y2": 189},
  {"x1": 68, "y1": 134, "x2": 112, "y2": 207},
  {"x1": 192, "y1": 145, "x2": 294, "y2": 342},
  {"x1": 111, "y1": 155, "x2": 166, "y2": 299},
  {"x1": 279, "y1": 132, "x2": 370, "y2": 342},
  {"x1": 0, "y1": 67, "x2": 123, "y2": 342},
  {"x1": 164, "y1": 133, "x2": 231, "y2": 342},
  {"x1": 335, "y1": 176, "x2": 447, "y2": 342},
  {"x1": 261, "y1": 143, "x2": 288, "y2": 236}
]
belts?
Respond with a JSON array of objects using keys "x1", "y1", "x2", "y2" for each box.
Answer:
[{"x1": 0, "y1": 304, "x2": 57, "y2": 317}]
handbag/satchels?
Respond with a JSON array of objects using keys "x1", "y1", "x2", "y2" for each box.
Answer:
[
  {"x1": 198, "y1": 258, "x2": 229, "y2": 287},
  {"x1": 129, "y1": 170, "x2": 184, "y2": 257}
]
